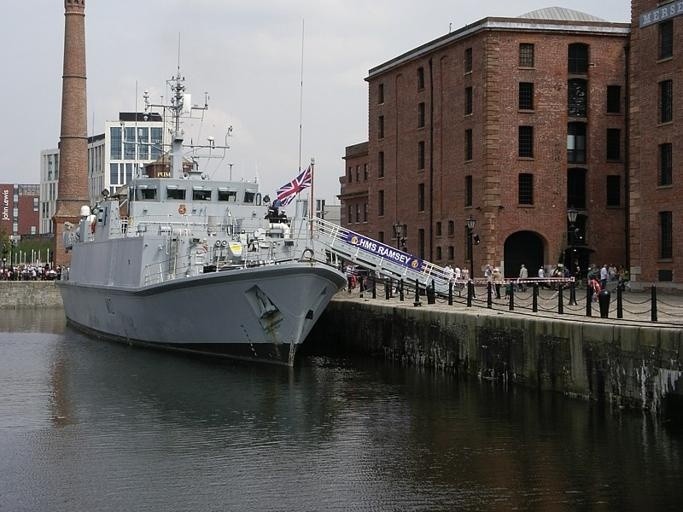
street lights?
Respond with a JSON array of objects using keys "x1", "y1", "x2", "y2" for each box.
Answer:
[
  {"x1": 393, "y1": 220, "x2": 402, "y2": 251},
  {"x1": 7, "y1": 234, "x2": 17, "y2": 270},
  {"x1": 563, "y1": 207, "x2": 579, "y2": 305},
  {"x1": 1, "y1": 244, "x2": 7, "y2": 273},
  {"x1": 463, "y1": 214, "x2": 479, "y2": 297}
]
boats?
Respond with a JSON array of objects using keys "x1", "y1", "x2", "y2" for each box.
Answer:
[{"x1": 48, "y1": 27, "x2": 349, "y2": 370}]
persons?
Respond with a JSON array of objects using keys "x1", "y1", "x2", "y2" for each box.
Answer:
[
  {"x1": 277, "y1": 210, "x2": 288, "y2": 237},
  {"x1": 429, "y1": 257, "x2": 627, "y2": 303},
  {"x1": 265, "y1": 209, "x2": 274, "y2": 237},
  {"x1": 0, "y1": 262, "x2": 70, "y2": 281}
]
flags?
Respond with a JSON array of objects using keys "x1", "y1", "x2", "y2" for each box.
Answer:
[{"x1": 276, "y1": 165, "x2": 311, "y2": 205}]
